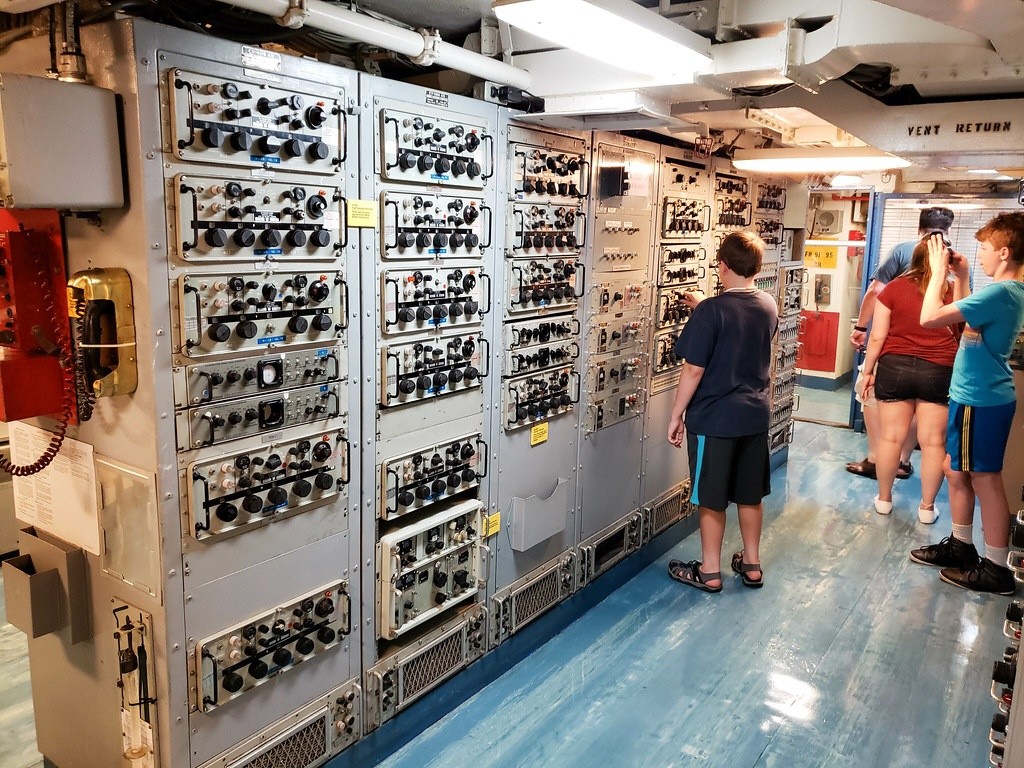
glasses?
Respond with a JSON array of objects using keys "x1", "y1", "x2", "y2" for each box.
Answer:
[{"x1": 714, "y1": 262, "x2": 730, "y2": 274}]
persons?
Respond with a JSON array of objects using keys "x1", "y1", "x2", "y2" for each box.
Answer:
[
  {"x1": 908, "y1": 209, "x2": 1024, "y2": 595},
  {"x1": 846, "y1": 206, "x2": 974, "y2": 481},
  {"x1": 666, "y1": 231, "x2": 780, "y2": 594},
  {"x1": 859, "y1": 232, "x2": 959, "y2": 524}
]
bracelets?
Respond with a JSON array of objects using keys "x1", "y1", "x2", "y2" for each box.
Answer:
[{"x1": 855, "y1": 325, "x2": 867, "y2": 332}]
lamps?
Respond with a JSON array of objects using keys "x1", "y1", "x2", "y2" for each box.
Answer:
[{"x1": 732, "y1": 144, "x2": 910, "y2": 174}]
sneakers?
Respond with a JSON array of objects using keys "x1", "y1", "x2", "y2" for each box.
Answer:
[
  {"x1": 939, "y1": 557, "x2": 1017, "y2": 595},
  {"x1": 909, "y1": 531, "x2": 980, "y2": 567},
  {"x1": 918, "y1": 504, "x2": 940, "y2": 524},
  {"x1": 873, "y1": 493, "x2": 894, "y2": 515}
]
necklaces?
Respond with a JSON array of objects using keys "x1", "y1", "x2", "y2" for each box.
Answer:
[{"x1": 724, "y1": 287, "x2": 757, "y2": 293}]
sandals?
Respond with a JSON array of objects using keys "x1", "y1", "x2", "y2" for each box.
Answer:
[
  {"x1": 731, "y1": 549, "x2": 763, "y2": 587},
  {"x1": 669, "y1": 559, "x2": 723, "y2": 593},
  {"x1": 895, "y1": 460, "x2": 914, "y2": 479},
  {"x1": 845, "y1": 458, "x2": 877, "y2": 480}
]
